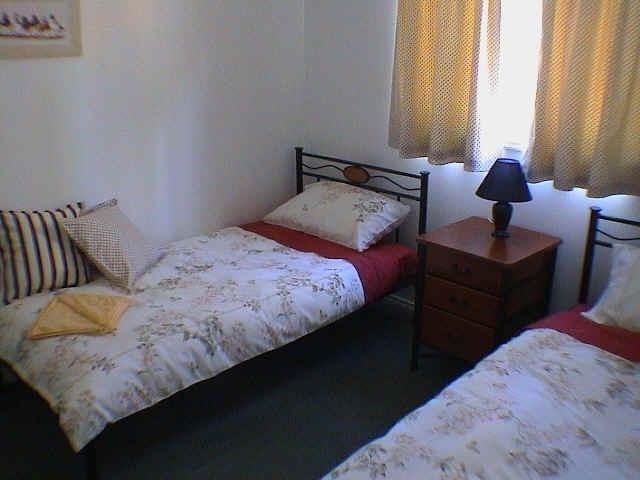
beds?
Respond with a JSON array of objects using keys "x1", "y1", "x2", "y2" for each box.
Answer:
[
  {"x1": 0, "y1": 147, "x2": 430, "y2": 480},
  {"x1": 321, "y1": 207, "x2": 639, "y2": 480}
]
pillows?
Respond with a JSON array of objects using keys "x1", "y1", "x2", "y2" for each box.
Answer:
[
  {"x1": 581, "y1": 244, "x2": 640, "y2": 333},
  {"x1": 0, "y1": 202, "x2": 93, "y2": 304},
  {"x1": 263, "y1": 182, "x2": 410, "y2": 253},
  {"x1": 62, "y1": 199, "x2": 162, "y2": 295}
]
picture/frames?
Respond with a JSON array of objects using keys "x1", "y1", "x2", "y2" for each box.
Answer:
[{"x1": 0, "y1": 0, "x2": 82, "y2": 58}]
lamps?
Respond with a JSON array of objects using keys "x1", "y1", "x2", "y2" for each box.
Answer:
[{"x1": 476, "y1": 158, "x2": 533, "y2": 238}]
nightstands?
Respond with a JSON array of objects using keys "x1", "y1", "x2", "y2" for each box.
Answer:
[{"x1": 410, "y1": 216, "x2": 560, "y2": 371}]
